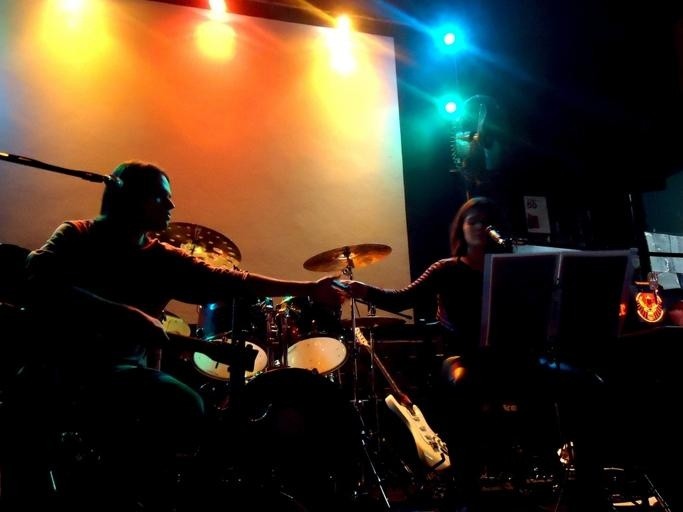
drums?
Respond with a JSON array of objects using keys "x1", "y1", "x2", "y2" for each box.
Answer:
[
  {"x1": 275, "y1": 296, "x2": 349, "y2": 374},
  {"x1": 193, "y1": 297, "x2": 270, "y2": 381},
  {"x1": 221, "y1": 368, "x2": 365, "y2": 512}
]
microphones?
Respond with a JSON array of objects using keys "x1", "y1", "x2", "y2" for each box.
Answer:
[
  {"x1": 106, "y1": 175, "x2": 124, "y2": 188},
  {"x1": 485, "y1": 224, "x2": 508, "y2": 250}
]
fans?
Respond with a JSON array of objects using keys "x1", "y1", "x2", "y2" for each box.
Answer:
[{"x1": 448, "y1": 92, "x2": 509, "y2": 187}]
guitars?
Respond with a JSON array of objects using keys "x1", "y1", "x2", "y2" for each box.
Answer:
[
  {"x1": 353, "y1": 327, "x2": 451, "y2": 472},
  {"x1": 0, "y1": 243, "x2": 258, "y2": 372}
]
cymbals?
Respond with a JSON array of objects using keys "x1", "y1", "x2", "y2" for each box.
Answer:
[
  {"x1": 148, "y1": 222, "x2": 241, "y2": 270},
  {"x1": 303, "y1": 244, "x2": 392, "y2": 272},
  {"x1": 341, "y1": 317, "x2": 405, "y2": 328}
]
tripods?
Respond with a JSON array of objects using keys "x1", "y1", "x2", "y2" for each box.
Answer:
[{"x1": 338, "y1": 268, "x2": 421, "y2": 512}]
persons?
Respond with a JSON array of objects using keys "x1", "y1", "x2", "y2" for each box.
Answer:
[
  {"x1": 336, "y1": 195, "x2": 498, "y2": 340},
  {"x1": 21, "y1": 161, "x2": 344, "y2": 414}
]
